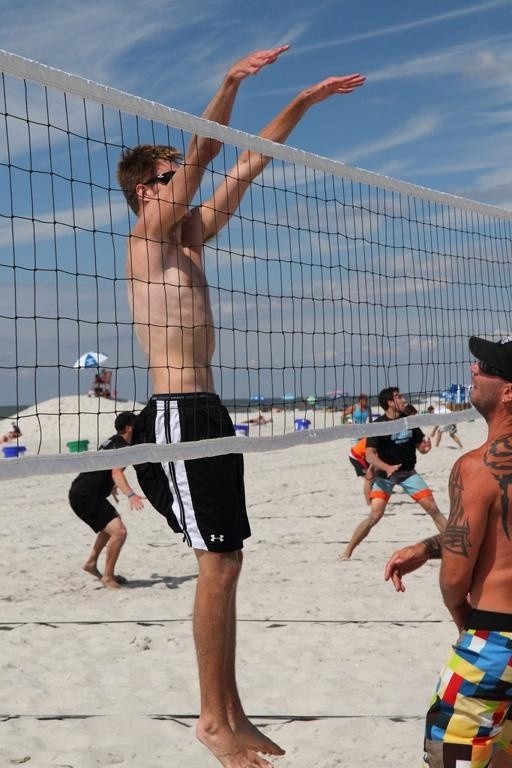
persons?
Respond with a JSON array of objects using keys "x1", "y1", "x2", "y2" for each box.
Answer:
[
  {"x1": 117, "y1": 43, "x2": 365, "y2": 768},
  {"x1": 427, "y1": 405, "x2": 462, "y2": 448},
  {"x1": 92, "y1": 372, "x2": 104, "y2": 392},
  {"x1": 385, "y1": 334, "x2": 512, "y2": 766},
  {"x1": 0, "y1": 433, "x2": 11, "y2": 443},
  {"x1": 11, "y1": 422, "x2": 22, "y2": 437},
  {"x1": 340, "y1": 387, "x2": 448, "y2": 561},
  {"x1": 341, "y1": 394, "x2": 374, "y2": 445},
  {"x1": 349, "y1": 404, "x2": 415, "y2": 508},
  {"x1": 67, "y1": 410, "x2": 146, "y2": 590}
]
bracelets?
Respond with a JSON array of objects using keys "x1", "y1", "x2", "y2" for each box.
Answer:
[{"x1": 128, "y1": 492, "x2": 135, "y2": 498}]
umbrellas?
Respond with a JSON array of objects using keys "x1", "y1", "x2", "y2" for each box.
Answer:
[
  {"x1": 73, "y1": 351, "x2": 110, "y2": 370},
  {"x1": 248, "y1": 389, "x2": 349, "y2": 404}
]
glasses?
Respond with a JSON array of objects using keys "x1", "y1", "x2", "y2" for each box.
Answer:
[{"x1": 141, "y1": 170, "x2": 175, "y2": 186}]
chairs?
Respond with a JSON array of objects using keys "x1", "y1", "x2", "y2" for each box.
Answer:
[{"x1": 95, "y1": 371, "x2": 112, "y2": 393}]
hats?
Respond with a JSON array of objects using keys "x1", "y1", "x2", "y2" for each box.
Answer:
[{"x1": 468, "y1": 332, "x2": 512, "y2": 378}]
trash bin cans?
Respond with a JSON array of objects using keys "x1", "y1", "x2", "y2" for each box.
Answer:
[
  {"x1": 295, "y1": 418, "x2": 311, "y2": 431},
  {"x1": 233, "y1": 424, "x2": 249, "y2": 437},
  {"x1": 67, "y1": 440, "x2": 89, "y2": 452},
  {"x1": 1, "y1": 447, "x2": 26, "y2": 459}
]
